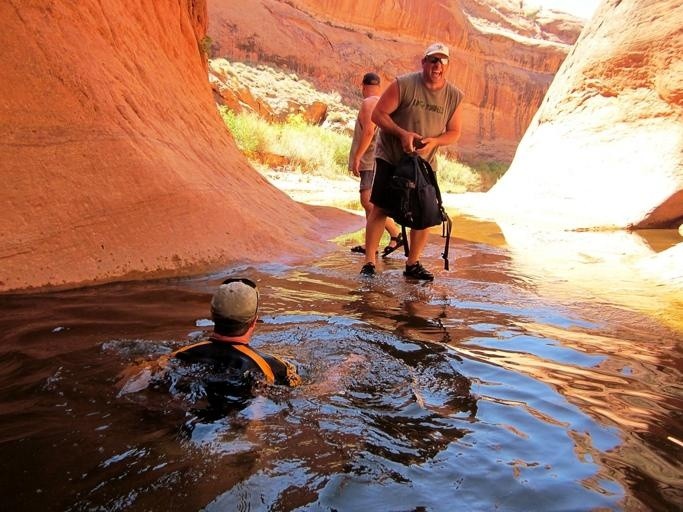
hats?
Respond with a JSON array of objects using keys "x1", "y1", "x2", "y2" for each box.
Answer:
[
  {"x1": 425, "y1": 43, "x2": 449, "y2": 58},
  {"x1": 210, "y1": 279, "x2": 260, "y2": 325},
  {"x1": 361, "y1": 72, "x2": 380, "y2": 85}
]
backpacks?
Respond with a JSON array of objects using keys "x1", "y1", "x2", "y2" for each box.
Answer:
[{"x1": 386, "y1": 150, "x2": 452, "y2": 270}]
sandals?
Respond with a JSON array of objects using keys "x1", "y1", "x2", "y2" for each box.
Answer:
[
  {"x1": 403, "y1": 260, "x2": 434, "y2": 280},
  {"x1": 381, "y1": 232, "x2": 404, "y2": 257},
  {"x1": 351, "y1": 244, "x2": 380, "y2": 255},
  {"x1": 360, "y1": 262, "x2": 377, "y2": 277}
]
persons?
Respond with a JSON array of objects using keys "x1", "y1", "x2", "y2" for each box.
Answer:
[
  {"x1": 347, "y1": 72, "x2": 407, "y2": 258},
  {"x1": 132, "y1": 273, "x2": 366, "y2": 405},
  {"x1": 355, "y1": 41, "x2": 467, "y2": 285}
]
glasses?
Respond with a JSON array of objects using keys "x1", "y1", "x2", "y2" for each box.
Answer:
[
  {"x1": 223, "y1": 277, "x2": 256, "y2": 288},
  {"x1": 427, "y1": 56, "x2": 449, "y2": 65}
]
